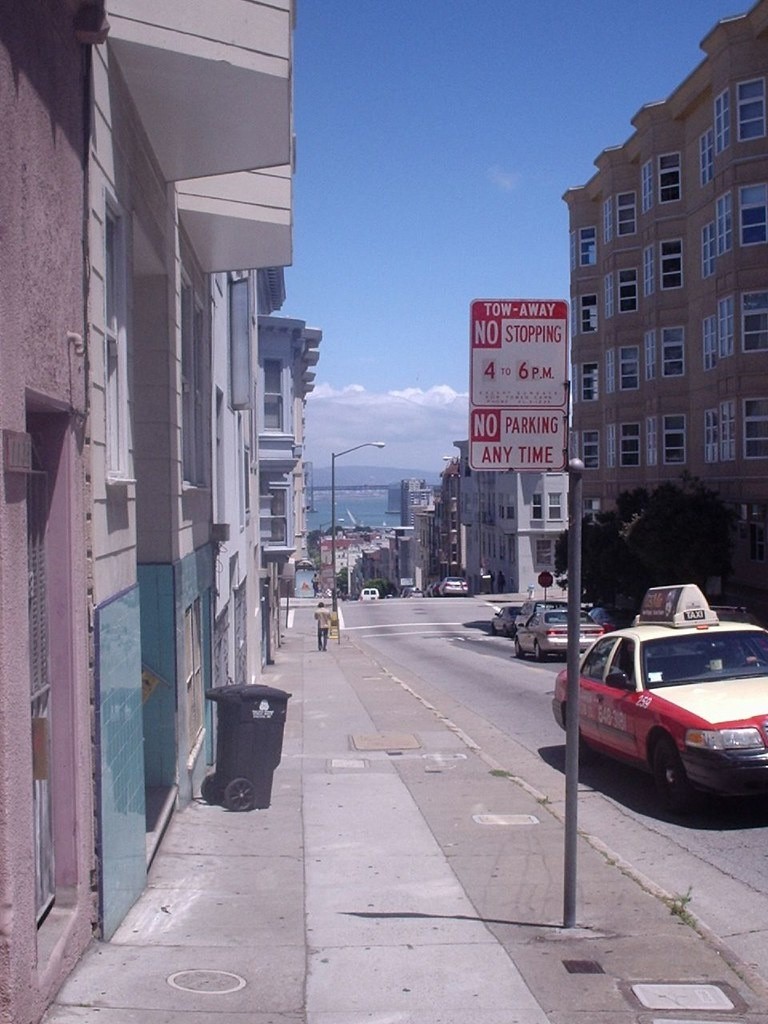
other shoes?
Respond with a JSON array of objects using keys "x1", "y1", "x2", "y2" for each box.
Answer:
[
  {"x1": 323, "y1": 649, "x2": 327, "y2": 651},
  {"x1": 319, "y1": 646, "x2": 321, "y2": 650}
]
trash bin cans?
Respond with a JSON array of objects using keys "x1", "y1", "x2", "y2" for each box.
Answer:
[{"x1": 199, "y1": 682, "x2": 294, "y2": 814}]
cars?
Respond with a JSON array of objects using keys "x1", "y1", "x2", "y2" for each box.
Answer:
[
  {"x1": 397, "y1": 576, "x2": 468, "y2": 599},
  {"x1": 513, "y1": 609, "x2": 605, "y2": 663},
  {"x1": 360, "y1": 588, "x2": 380, "y2": 601},
  {"x1": 491, "y1": 606, "x2": 523, "y2": 638},
  {"x1": 551, "y1": 584, "x2": 768, "y2": 802}
]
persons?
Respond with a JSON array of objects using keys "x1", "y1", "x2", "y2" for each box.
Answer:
[
  {"x1": 726, "y1": 637, "x2": 757, "y2": 668},
  {"x1": 311, "y1": 573, "x2": 319, "y2": 598},
  {"x1": 314, "y1": 602, "x2": 331, "y2": 652},
  {"x1": 487, "y1": 570, "x2": 495, "y2": 594},
  {"x1": 497, "y1": 571, "x2": 506, "y2": 593}
]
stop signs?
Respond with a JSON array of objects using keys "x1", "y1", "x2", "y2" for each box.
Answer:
[{"x1": 538, "y1": 571, "x2": 553, "y2": 588}]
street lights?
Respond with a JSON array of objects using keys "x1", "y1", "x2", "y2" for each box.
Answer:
[
  {"x1": 331, "y1": 441, "x2": 386, "y2": 613},
  {"x1": 442, "y1": 456, "x2": 485, "y2": 571},
  {"x1": 318, "y1": 517, "x2": 345, "y2": 577}
]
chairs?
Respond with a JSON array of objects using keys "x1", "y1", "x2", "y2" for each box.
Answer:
[{"x1": 708, "y1": 638, "x2": 736, "y2": 667}]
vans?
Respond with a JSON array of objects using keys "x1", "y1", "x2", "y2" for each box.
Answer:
[{"x1": 513, "y1": 600, "x2": 568, "y2": 632}]
